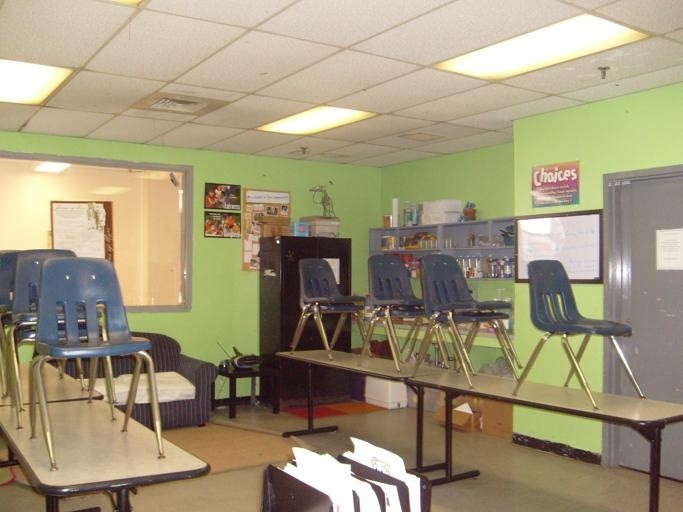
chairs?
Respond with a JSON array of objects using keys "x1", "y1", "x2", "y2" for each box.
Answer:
[
  {"x1": 1, "y1": 248, "x2": 119, "y2": 432},
  {"x1": 289, "y1": 253, "x2": 524, "y2": 390},
  {"x1": 27, "y1": 255, "x2": 166, "y2": 474},
  {"x1": 509, "y1": 257, "x2": 648, "y2": 413}
]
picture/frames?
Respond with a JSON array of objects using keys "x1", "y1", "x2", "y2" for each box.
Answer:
[
  {"x1": 515, "y1": 207, "x2": 603, "y2": 285},
  {"x1": 202, "y1": 181, "x2": 241, "y2": 211},
  {"x1": 202, "y1": 210, "x2": 242, "y2": 239}
]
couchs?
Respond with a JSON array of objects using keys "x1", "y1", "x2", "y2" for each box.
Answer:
[{"x1": 28, "y1": 330, "x2": 219, "y2": 432}]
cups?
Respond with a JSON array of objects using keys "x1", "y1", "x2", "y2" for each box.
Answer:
[{"x1": 418, "y1": 238, "x2": 454, "y2": 249}]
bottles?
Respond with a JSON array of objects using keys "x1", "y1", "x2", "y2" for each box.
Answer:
[
  {"x1": 408, "y1": 257, "x2": 416, "y2": 279},
  {"x1": 456, "y1": 253, "x2": 483, "y2": 281},
  {"x1": 487, "y1": 257, "x2": 514, "y2": 278},
  {"x1": 402, "y1": 198, "x2": 414, "y2": 230}
]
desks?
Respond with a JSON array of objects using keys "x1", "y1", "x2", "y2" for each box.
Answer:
[
  {"x1": 0, "y1": 397, "x2": 211, "y2": 512},
  {"x1": 0, "y1": 359, "x2": 104, "y2": 469},
  {"x1": 273, "y1": 346, "x2": 448, "y2": 476},
  {"x1": 405, "y1": 370, "x2": 683, "y2": 512}
]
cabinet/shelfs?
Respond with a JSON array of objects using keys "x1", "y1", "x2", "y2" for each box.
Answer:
[
  {"x1": 258, "y1": 235, "x2": 353, "y2": 409},
  {"x1": 368, "y1": 216, "x2": 514, "y2": 281}
]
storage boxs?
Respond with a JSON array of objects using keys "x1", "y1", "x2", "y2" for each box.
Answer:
[
  {"x1": 299, "y1": 216, "x2": 340, "y2": 238},
  {"x1": 361, "y1": 374, "x2": 512, "y2": 440},
  {"x1": 255, "y1": 215, "x2": 309, "y2": 239},
  {"x1": 420, "y1": 200, "x2": 460, "y2": 226}
]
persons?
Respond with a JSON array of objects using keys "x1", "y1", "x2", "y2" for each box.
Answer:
[
  {"x1": 204, "y1": 183, "x2": 231, "y2": 209},
  {"x1": 205, "y1": 214, "x2": 240, "y2": 237}
]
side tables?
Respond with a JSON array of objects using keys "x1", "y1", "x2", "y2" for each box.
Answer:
[{"x1": 210, "y1": 363, "x2": 280, "y2": 419}]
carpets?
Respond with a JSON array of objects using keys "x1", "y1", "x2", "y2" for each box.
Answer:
[
  {"x1": 150, "y1": 417, "x2": 316, "y2": 476},
  {"x1": 282, "y1": 400, "x2": 385, "y2": 421}
]
